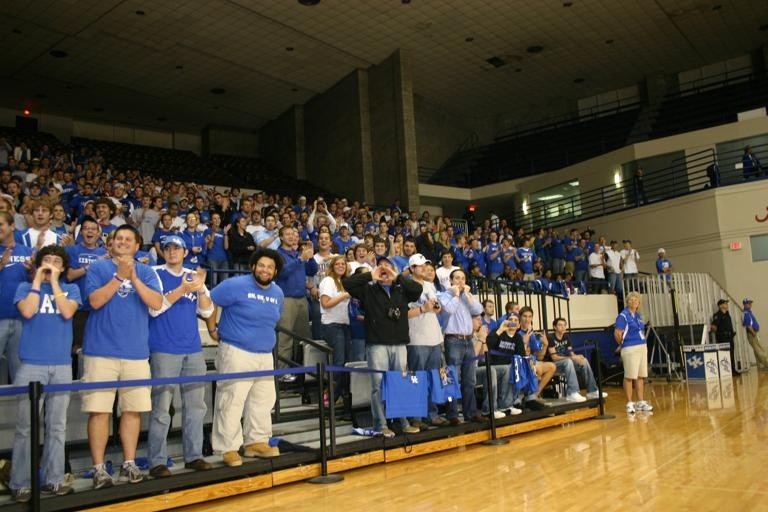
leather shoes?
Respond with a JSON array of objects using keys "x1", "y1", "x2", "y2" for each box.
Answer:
[
  {"x1": 243, "y1": 442, "x2": 281, "y2": 458},
  {"x1": 222, "y1": 450, "x2": 243, "y2": 468}
]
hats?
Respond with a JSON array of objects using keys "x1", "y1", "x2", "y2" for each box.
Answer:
[
  {"x1": 407, "y1": 253, "x2": 433, "y2": 268},
  {"x1": 83, "y1": 200, "x2": 95, "y2": 209},
  {"x1": 29, "y1": 181, "x2": 42, "y2": 189},
  {"x1": 610, "y1": 239, "x2": 620, "y2": 244},
  {"x1": 112, "y1": 182, "x2": 125, "y2": 190},
  {"x1": 742, "y1": 296, "x2": 754, "y2": 305},
  {"x1": 658, "y1": 247, "x2": 666, "y2": 254},
  {"x1": 179, "y1": 197, "x2": 189, "y2": 202},
  {"x1": 49, "y1": 181, "x2": 65, "y2": 193},
  {"x1": 342, "y1": 206, "x2": 352, "y2": 212},
  {"x1": 717, "y1": 299, "x2": 729, "y2": 305},
  {"x1": 299, "y1": 196, "x2": 307, "y2": 200},
  {"x1": 376, "y1": 256, "x2": 395, "y2": 267},
  {"x1": 508, "y1": 312, "x2": 521, "y2": 320},
  {"x1": 622, "y1": 239, "x2": 632, "y2": 243},
  {"x1": 159, "y1": 234, "x2": 187, "y2": 251}
]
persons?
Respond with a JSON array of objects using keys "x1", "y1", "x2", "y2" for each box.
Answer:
[
  {"x1": 710, "y1": 298, "x2": 740, "y2": 376},
  {"x1": 743, "y1": 144, "x2": 760, "y2": 178},
  {"x1": 743, "y1": 297, "x2": 768, "y2": 370},
  {"x1": 1, "y1": 136, "x2": 653, "y2": 503},
  {"x1": 655, "y1": 247, "x2": 673, "y2": 293}
]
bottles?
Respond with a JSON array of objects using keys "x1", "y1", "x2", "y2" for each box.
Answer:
[{"x1": 324, "y1": 389, "x2": 330, "y2": 410}]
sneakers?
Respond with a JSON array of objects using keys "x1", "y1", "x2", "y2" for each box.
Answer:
[
  {"x1": 586, "y1": 390, "x2": 609, "y2": 399},
  {"x1": 184, "y1": 459, "x2": 212, "y2": 471},
  {"x1": 498, "y1": 405, "x2": 523, "y2": 416},
  {"x1": 514, "y1": 395, "x2": 551, "y2": 409},
  {"x1": 636, "y1": 400, "x2": 654, "y2": 412},
  {"x1": 149, "y1": 464, "x2": 171, "y2": 478},
  {"x1": 374, "y1": 428, "x2": 396, "y2": 439},
  {"x1": 449, "y1": 418, "x2": 461, "y2": 427},
  {"x1": 565, "y1": 391, "x2": 586, "y2": 402},
  {"x1": 625, "y1": 401, "x2": 636, "y2": 413},
  {"x1": 401, "y1": 425, "x2": 421, "y2": 434},
  {"x1": 430, "y1": 417, "x2": 450, "y2": 428},
  {"x1": 464, "y1": 413, "x2": 492, "y2": 423},
  {"x1": 410, "y1": 421, "x2": 429, "y2": 432},
  {"x1": 15, "y1": 487, "x2": 32, "y2": 503},
  {"x1": 757, "y1": 362, "x2": 765, "y2": 369},
  {"x1": 732, "y1": 367, "x2": 741, "y2": 377},
  {"x1": 118, "y1": 462, "x2": 144, "y2": 484},
  {"x1": 480, "y1": 409, "x2": 507, "y2": 420},
  {"x1": 91, "y1": 464, "x2": 113, "y2": 490},
  {"x1": 39, "y1": 481, "x2": 74, "y2": 496},
  {"x1": 321, "y1": 389, "x2": 332, "y2": 409},
  {"x1": 277, "y1": 372, "x2": 299, "y2": 383}
]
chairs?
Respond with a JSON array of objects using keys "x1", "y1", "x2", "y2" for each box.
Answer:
[{"x1": 0, "y1": 333, "x2": 572, "y2": 481}]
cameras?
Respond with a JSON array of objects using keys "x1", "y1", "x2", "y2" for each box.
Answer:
[
  {"x1": 433, "y1": 300, "x2": 440, "y2": 310},
  {"x1": 388, "y1": 304, "x2": 401, "y2": 320},
  {"x1": 506, "y1": 322, "x2": 518, "y2": 328}
]
